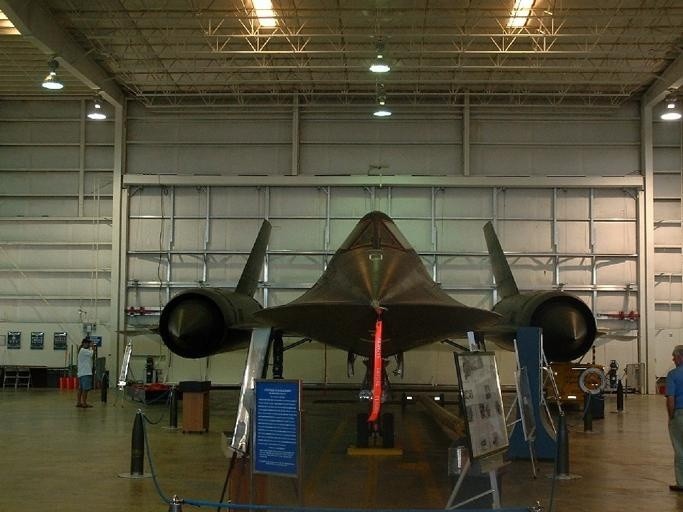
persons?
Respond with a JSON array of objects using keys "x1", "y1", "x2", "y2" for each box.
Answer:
[
  {"x1": 73, "y1": 338, "x2": 97, "y2": 408},
  {"x1": 662, "y1": 344, "x2": 683, "y2": 492}
]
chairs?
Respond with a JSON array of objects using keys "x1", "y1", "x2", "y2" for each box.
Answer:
[{"x1": 2, "y1": 364, "x2": 34, "y2": 393}]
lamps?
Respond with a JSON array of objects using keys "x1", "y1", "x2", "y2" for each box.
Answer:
[
  {"x1": 660, "y1": 93, "x2": 681, "y2": 120},
  {"x1": 373, "y1": 91, "x2": 392, "y2": 118},
  {"x1": 369, "y1": 39, "x2": 391, "y2": 74},
  {"x1": 87, "y1": 90, "x2": 107, "y2": 120},
  {"x1": 40, "y1": 56, "x2": 64, "y2": 90}
]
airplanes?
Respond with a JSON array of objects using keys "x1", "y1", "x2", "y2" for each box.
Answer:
[{"x1": 115, "y1": 210, "x2": 641, "y2": 449}]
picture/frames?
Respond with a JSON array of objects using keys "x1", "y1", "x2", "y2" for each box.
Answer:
[
  {"x1": 90, "y1": 337, "x2": 102, "y2": 347},
  {"x1": 31, "y1": 332, "x2": 44, "y2": 349},
  {"x1": 53, "y1": 332, "x2": 67, "y2": 350},
  {"x1": 7, "y1": 332, "x2": 21, "y2": 349}
]
